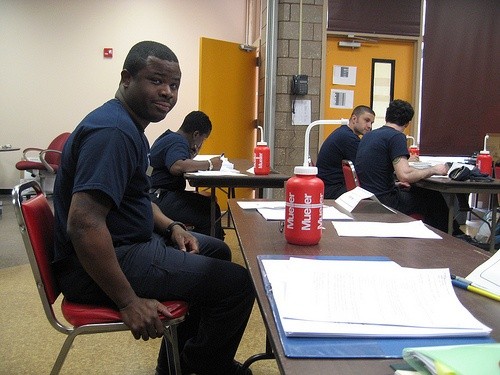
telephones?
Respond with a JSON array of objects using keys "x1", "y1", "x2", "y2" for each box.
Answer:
[{"x1": 291, "y1": 74, "x2": 308, "y2": 95}]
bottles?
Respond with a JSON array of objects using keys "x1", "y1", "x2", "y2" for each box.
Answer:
[
  {"x1": 253, "y1": 141, "x2": 271, "y2": 175},
  {"x1": 476, "y1": 151, "x2": 493, "y2": 177},
  {"x1": 285, "y1": 166, "x2": 325, "y2": 246},
  {"x1": 408, "y1": 145, "x2": 419, "y2": 155}
]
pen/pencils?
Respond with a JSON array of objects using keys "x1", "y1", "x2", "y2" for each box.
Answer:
[
  {"x1": 451, "y1": 274, "x2": 500, "y2": 302},
  {"x1": 220, "y1": 153, "x2": 224, "y2": 158}
]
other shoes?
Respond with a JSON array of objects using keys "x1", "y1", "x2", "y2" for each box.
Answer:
[
  {"x1": 155, "y1": 365, "x2": 197, "y2": 375},
  {"x1": 227, "y1": 359, "x2": 252, "y2": 375},
  {"x1": 455, "y1": 229, "x2": 466, "y2": 238}
]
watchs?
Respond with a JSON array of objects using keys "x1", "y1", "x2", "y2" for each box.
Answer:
[
  {"x1": 166, "y1": 221, "x2": 187, "y2": 237},
  {"x1": 207, "y1": 159, "x2": 214, "y2": 170}
]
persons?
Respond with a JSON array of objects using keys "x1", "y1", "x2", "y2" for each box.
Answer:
[
  {"x1": 315, "y1": 105, "x2": 375, "y2": 200},
  {"x1": 146, "y1": 110, "x2": 226, "y2": 243},
  {"x1": 355, "y1": 98, "x2": 477, "y2": 247},
  {"x1": 50, "y1": 38, "x2": 259, "y2": 375}
]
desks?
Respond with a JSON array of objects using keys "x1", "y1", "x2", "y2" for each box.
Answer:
[
  {"x1": 184, "y1": 159, "x2": 289, "y2": 238},
  {"x1": 414, "y1": 178, "x2": 500, "y2": 253},
  {"x1": 226, "y1": 198, "x2": 500, "y2": 375}
]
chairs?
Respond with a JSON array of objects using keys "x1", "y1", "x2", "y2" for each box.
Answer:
[
  {"x1": 16, "y1": 132, "x2": 71, "y2": 200},
  {"x1": 12, "y1": 181, "x2": 189, "y2": 375},
  {"x1": 342, "y1": 159, "x2": 424, "y2": 221}
]
